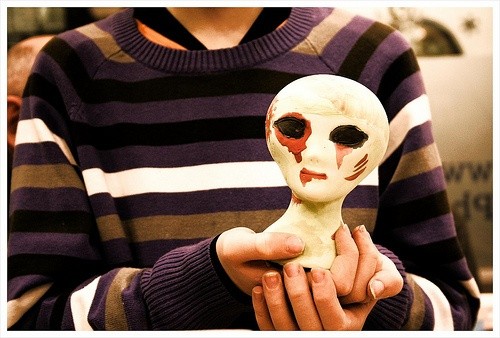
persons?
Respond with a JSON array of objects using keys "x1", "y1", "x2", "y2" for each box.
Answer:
[{"x1": 7, "y1": 6, "x2": 485, "y2": 331}]
